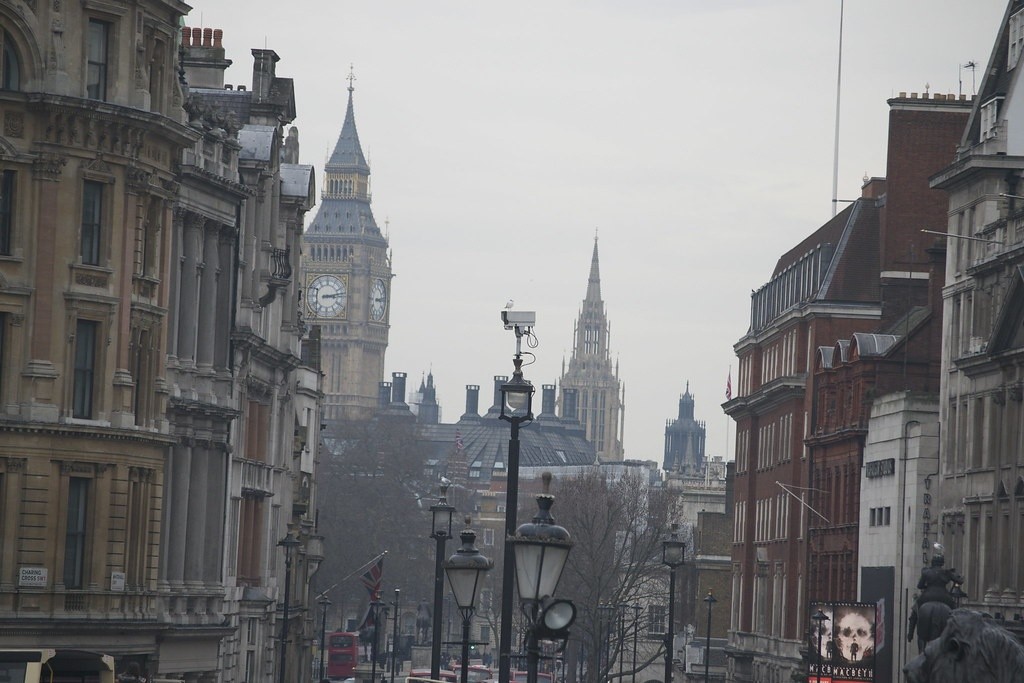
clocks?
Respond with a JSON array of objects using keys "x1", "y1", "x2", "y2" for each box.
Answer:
[
  {"x1": 369, "y1": 278, "x2": 388, "y2": 322},
  {"x1": 306, "y1": 274, "x2": 347, "y2": 319}
]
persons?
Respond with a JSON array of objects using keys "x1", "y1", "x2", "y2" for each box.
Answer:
[
  {"x1": 907, "y1": 553, "x2": 965, "y2": 642},
  {"x1": 825, "y1": 637, "x2": 832, "y2": 659},
  {"x1": 441, "y1": 649, "x2": 493, "y2": 670},
  {"x1": 849, "y1": 639, "x2": 859, "y2": 661}
]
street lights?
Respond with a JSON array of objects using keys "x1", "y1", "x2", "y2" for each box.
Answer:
[
  {"x1": 703, "y1": 588, "x2": 719, "y2": 683},
  {"x1": 596, "y1": 598, "x2": 606, "y2": 683},
  {"x1": 661, "y1": 523, "x2": 687, "y2": 682},
  {"x1": 318, "y1": 596, "x2": 333, "y2": 683},
  {"x1": 429, "y1": 485, "x2": 457, "y2": 680},
  {"x1": 498, "y1": 471, "x2": 576, "y2": 683},
  {"x1": 630, "y1": 602, "x2": 644, "y2": 683},
  {"x1": 275, "y1": 523, "x2": 299, "y2": 683},
  {"x1": 498, "y1": 355, "x2": 536, "y2": 683},
  {"x1": 618, "y1": 599, "x2": 630, "y2": 683},
  {"x1": 811, "y1": 609, "x2": 830, "y2": 683},
  {"x1": 370, "y1": 596, "x2": 386, "y2": 683},
  {"x1": 441, "y1": 515, "x2": 494, "y2": 683},
  {"x1": 605, "y1": 598, "x2": 615, "y2": 683}
]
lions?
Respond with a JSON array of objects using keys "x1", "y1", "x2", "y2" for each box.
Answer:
[{"x1": 902, "y1": 607, "x2": 1024, "y2": 683}]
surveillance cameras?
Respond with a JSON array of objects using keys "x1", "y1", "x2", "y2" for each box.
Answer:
[{"x1": 501, "y1": 311, "x2": 536, "y2": 327}]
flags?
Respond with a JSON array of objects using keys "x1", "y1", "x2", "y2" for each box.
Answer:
[{"x1": 361, "y1": 556, "x2": 384, "y2": 602}]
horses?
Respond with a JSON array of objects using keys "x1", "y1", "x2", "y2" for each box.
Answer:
[{"x1": 919, "y1": 600, "x2": 952, "y2": 641}]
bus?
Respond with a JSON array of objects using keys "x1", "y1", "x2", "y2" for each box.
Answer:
[
  {"x1": 409, "y1": 669, "x2": 457, "y2": 683},
  {"x1": 452, "y1": 665, "x2": 492, "y2": 683},
  {"x1": 509, "y1": 671, "x2": 553, "y2": 683},
  {"x1": 329, "y1": 632, "x2": 360, "y2": 680}
]
